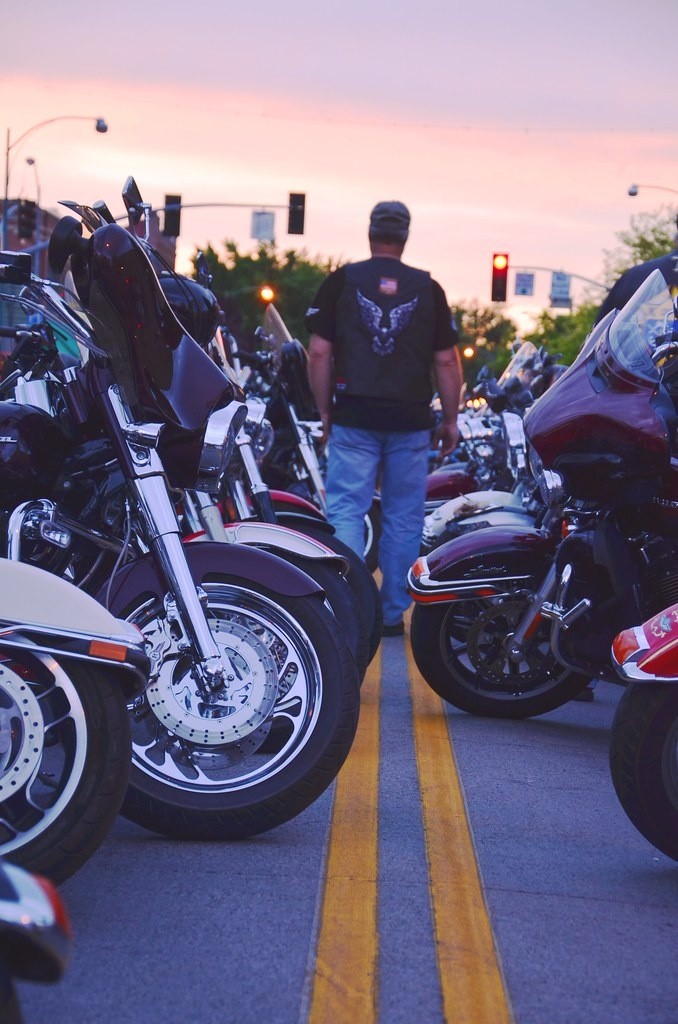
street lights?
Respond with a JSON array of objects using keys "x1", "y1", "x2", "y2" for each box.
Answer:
[{"x1": 4, "y1": 114, "x2": 107, "y2": 201}]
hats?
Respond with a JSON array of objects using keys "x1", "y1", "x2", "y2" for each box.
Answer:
[{"x1": 371, "y1": 201, "x2": 410, "y2": 229}]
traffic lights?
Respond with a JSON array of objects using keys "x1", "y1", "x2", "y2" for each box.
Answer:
[{"x1": 491, "y1": 252, "x2": 512, "y2": 302}]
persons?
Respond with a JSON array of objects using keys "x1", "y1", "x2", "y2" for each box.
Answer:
[
  {"x1": 594, "y1": 212, "x2": 678, "y2": 327},
  {"x1": 303, "y1": 201, "x2": 462, "y2": 637}
]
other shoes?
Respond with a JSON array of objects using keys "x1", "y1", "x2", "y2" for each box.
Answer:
[{"x1": 382, "y1": 623, "x2": 405, "y2": 636}]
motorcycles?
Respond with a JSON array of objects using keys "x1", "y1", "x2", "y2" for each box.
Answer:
[{"x1": 0, "y1": 198, "x2": 678, "y2": 1023}]
